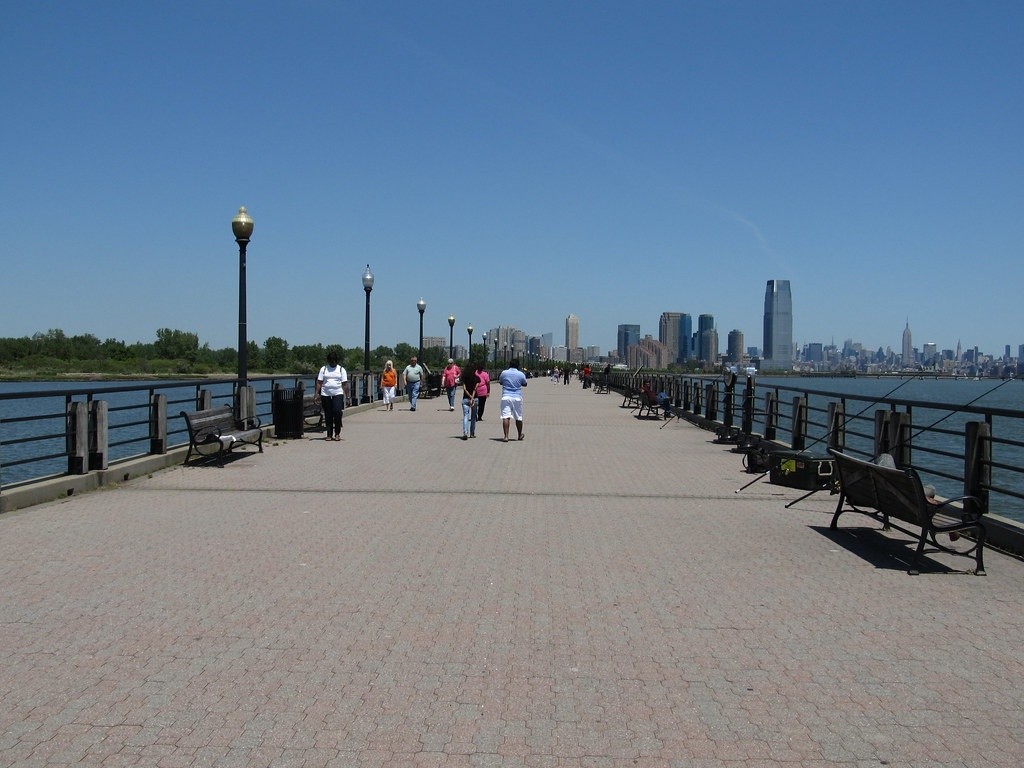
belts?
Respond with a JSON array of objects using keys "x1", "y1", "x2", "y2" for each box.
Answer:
[{"x1": 407, "y1": 380, "x2": 420, "y2": 384}]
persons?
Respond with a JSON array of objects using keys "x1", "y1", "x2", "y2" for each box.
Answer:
[
  {"x1": 497, "y1": 367, "x2": 508, "y2": 383},
  {"x1": 442, "y1": 358, "x2": 462, "y2": 410},
  {"x1": 468, "y1": 363, "x2": 490, "y2": 422},
  {"x1": 402, "y1": 355, "x2": 423, "y2": 411},
  {"x1": 437, "y1": 367, "x2": 444, "y2": 396},
  {"x1": 457, "y1": 365, "x2": 481, "y2": 440},
  {"x1": 379, "y1": 359, "x2": 397, "y2": 412},
  {"x1": 583, "y1": 365, "x2": 592, "y2": 389},
  {"x1": 640, "y1": 380, "x2": 673, "y2": 420},
  {"x1": 313, "y1": 352, "x2": 352, "y2": 441},
  {"x1": 874, "y1": 453, "x2": 935, "y2": 499},
  {"x1": 499, "y1": 359, "x2": 527, "y2": 442},
  {"x1": 546, "y1": 365, "x2": 572, "y2": 385}
]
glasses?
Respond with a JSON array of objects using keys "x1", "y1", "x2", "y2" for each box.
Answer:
[{"x1": 448, "y1": 363, "x2": 453, "y2": 365}]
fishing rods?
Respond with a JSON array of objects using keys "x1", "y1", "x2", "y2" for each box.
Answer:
[
  {"x1": 630, "y1": 376, "x2": 678, "y2": 413},
  {"x1": 734, "y1": 361, "x2": 937, "y2": 495},
  {"x1": 659, "y1": 358, "x2": 741, "y2": 430},
  {"x1": 784, "y1": 370, "x2": 1024, "y2": 511}
]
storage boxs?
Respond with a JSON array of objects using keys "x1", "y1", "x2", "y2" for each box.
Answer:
[{"x1": 769, "y1": 448, "x2": 838, "y2": 491}]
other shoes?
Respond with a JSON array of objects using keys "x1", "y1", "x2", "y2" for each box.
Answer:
[
  {"x1": 664, "y1": 413, "x2": 676, "y2": 418},
  {"x1": 334, "y1": 433, "x2": 340, "y2": 441},
  {"x1": 478, "y1": 416, "x2": 482, "y2": 421},
  {"x1": 325, "y1": 435, "x2": 333, "y2": 440},
  {"x1": 410, "y1": 407, "x2": 416, "y2": 411},
  {"x1": 450, "y1": 406, "x2": 454, "y2": 411},
  {"x1": 518, "y1": 434, "x2": 524, "y2": 440},
  {"x1": 462, "y1": 435, "x2": 468, "y2": 440},
  {"x1": 502, "y1": 436, "x2": 509, "y2": 442},
  {"x1": 390, "y1": 403, "x2": 394, "y2": 409},
  {"x1": 469, "y1": 435, "x2": 476, "y2": 438}
]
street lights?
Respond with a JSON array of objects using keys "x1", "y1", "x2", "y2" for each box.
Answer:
[
  {"x1": 467, "y1": 322, "x2": 473, "y2": 359},
  {"x1": 494, "y1": 338, "x2": 498, "y2": 370},
  {"x1": 362, "y1": 264, "x2": 373, "y2": 404},
  {"x1": 504, "y1": 342, "x2": 507, "y2": 362},
  {"x1": 448, "y1": 314, "x2": 457, "y2": 359},
  {"x1": 417, "y1": 297, "x2": 426, "y2": 368},
  {"x1": 511, "y1": 345, "x2": 545, "y2": 371},
  {"x1": 232, "y1": 207, "x2": 253, "y2": 396},
  {"x1": 482, "y1": 332, "x2": 487, "y2": 360}
]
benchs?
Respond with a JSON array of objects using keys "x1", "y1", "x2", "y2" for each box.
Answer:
[
  {"x1": 419, "y1": 385, "x2": 446, "y2": 398},
  {"x1": 180, "y1": 404, "x2": 263, "y2": 468},
  {"x1": 303, "y1": 395, "x2": 344, "y2": 432},
  {"x1": 827, "y1": 447, "x2": 987, "y2": 574},
  {"x1": 622, "y1": 383, "x2": 662, "y2": 419},
  {"x1": 593, "y1": 378, "x2": 610, "y2": 394}
]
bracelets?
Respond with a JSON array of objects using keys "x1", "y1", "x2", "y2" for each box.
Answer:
[{"x1": 346, "y1": 397, "x2": 350, "y2": 399}]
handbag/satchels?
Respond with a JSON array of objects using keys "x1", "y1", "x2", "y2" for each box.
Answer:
[{"x1": 455, "y1": 377, "x2": 461, "y2": 383}]
